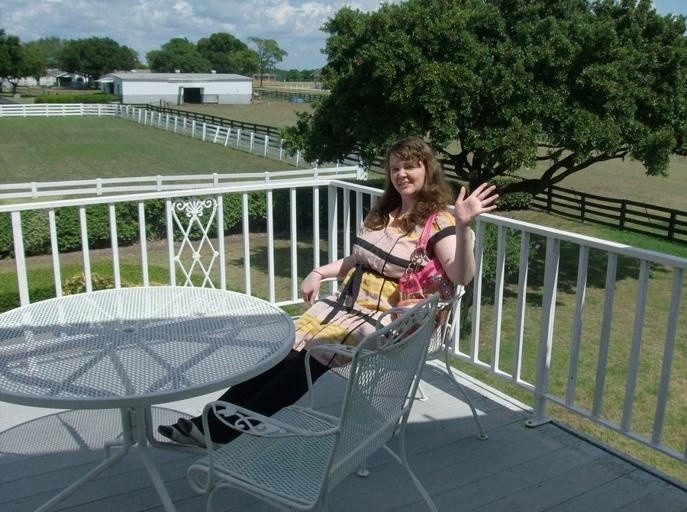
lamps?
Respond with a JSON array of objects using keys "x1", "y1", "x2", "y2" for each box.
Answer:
[
  {"x1": 185, "y1": 292, "x2": 438, "y2": 512},
  {"x1": 312, "y1": 227, "x2": 489, "y2": 476}
]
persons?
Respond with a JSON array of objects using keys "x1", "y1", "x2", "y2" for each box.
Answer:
[{"x1": 158, "y1": 137, "x2": 499, "y2": 449}]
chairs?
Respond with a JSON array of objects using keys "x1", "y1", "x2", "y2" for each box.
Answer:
[
  {"x1": 178, "y1": 418, "x2": 224, "y2": 451},
  {"x1": 157, "y1": 423, "x2": 207, "y2": 451}
]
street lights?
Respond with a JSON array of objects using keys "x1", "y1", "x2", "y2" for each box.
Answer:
[{"x1": 395, "y1": 212, "x2": 454, "y2": 336}]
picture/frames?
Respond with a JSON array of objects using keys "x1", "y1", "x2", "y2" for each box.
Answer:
[{"x1": 1, "y1": 284, "x2": 293, "y2": 512}]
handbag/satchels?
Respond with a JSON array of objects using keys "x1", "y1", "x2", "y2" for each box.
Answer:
[{"x1": 313, "y1": 269, "x2": 326, "y2": 279}]
